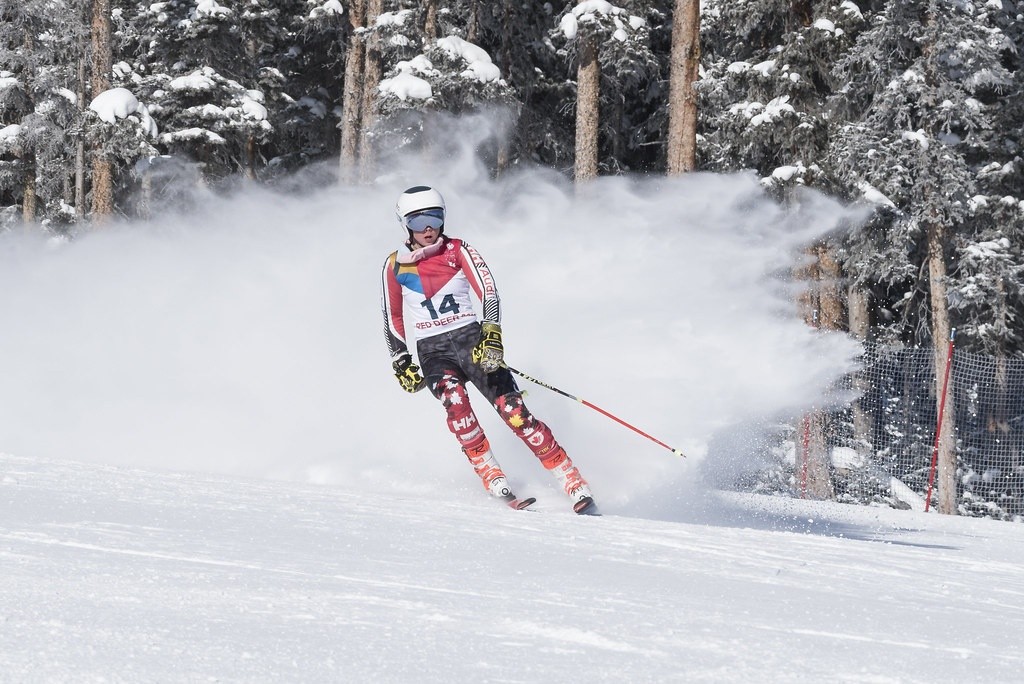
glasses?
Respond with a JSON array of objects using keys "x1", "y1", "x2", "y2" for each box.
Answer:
[{"x1": 406, "y1": 208, "x2": 444, "y2": 232}]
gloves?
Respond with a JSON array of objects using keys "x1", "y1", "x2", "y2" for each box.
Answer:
[
  {"x1": 472, "y1": 322, "x2": 504, "y2": 374},
  {"x1": 393, "y1": 353, "x2": 427, "y2": 393}
]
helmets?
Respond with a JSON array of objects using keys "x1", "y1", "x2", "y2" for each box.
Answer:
[{"x1": 396, "y1": 184, "x2": 445, "y2": 217}]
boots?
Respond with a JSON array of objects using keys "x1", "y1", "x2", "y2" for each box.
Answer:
[
  {"x1": 536, "y1": 441, "x2": 593, "y2": 513},
  {"x1": 461, "y1": 432, "x2": 536, "y2": 510}
]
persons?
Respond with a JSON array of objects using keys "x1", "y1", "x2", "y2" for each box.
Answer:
[{"x1": 379, "y1": 187, "x2": 592, "y2": 512}]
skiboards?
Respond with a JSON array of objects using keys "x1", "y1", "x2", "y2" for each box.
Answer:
[{"x1": 500, "y1": 493, "x2": 598, "y2": 516}]
